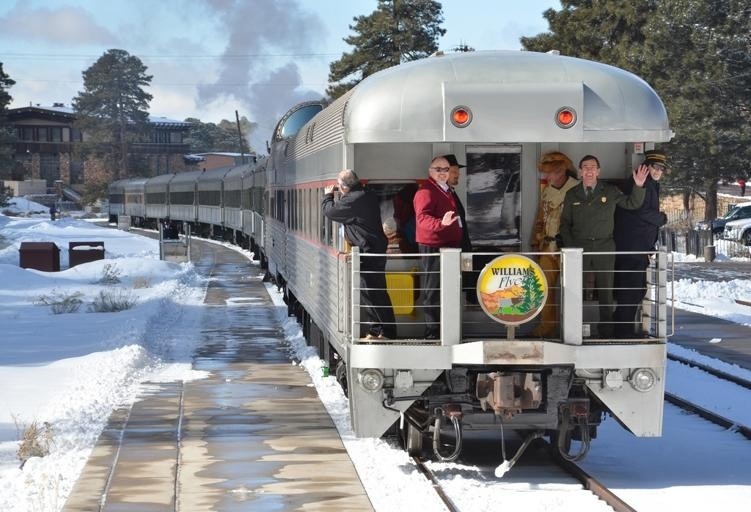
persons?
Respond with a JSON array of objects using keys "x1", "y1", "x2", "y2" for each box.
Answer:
[
  {"x1": 436, "y1": 154, "x2": 473, "y2": 292},
  {"x1": 612, "y1": 150, "x2": 671, "y2": 338},
  {"x1": 527, "y1": 151, "x2": 582, "y2": 338},
  {"x1": 414, "y1": 156, "x2": 463, "y2": 341},
  {"x1": 50, "y1": 204, "x2": 61, "y2": 220},
  {"x1": 322, "y1": 170, "x2": 396, "y2": 343},
  {"x1": 364, "y1": 182, "x2": 421, "y2": 260},
  {"x1": 558, "y1": 155, "x2": 650, "y2": 338},
  {"x1": 738, "y1": 177, "x2": 748, "y2": 196}
]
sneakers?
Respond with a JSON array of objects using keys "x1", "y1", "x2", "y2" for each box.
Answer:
[
  {"x1": 360, "y1": 334, "x2": 392, "y2": 343},
  {"x1": 529, "y1": 324, "x2": 557, "y2": 338}
]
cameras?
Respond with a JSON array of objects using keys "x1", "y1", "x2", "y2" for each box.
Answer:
[{"x1": 334, "y1": 186, "x2": 340, "y2": 191}]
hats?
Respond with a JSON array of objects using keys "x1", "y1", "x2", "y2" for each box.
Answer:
[
  {"x1": 443, "y1": 154, "x2": 467, "y2": 168},
  {"x1": 644, "y1": 150, "x2": 672, "y2": 168}
]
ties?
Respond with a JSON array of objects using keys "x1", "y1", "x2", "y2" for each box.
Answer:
[{"x1": 587, "y1": 185, "x2": 592, "y2": 201}]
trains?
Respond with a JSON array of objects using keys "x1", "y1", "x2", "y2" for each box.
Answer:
[{"x1": 109, "y1": 48, "x2": 676, "y2": 466}]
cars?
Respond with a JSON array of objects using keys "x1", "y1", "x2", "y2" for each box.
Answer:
[{"x1": 696, "y1": 203, "x2": 751, "y2": 246}]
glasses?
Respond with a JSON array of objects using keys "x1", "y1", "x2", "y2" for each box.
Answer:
[
  {"x1": 652, "y1": 164, "x2": 666, "y2": 171},
  {"x1": 430, "y1": 166, "x2": 449, "y2": 172}
]
